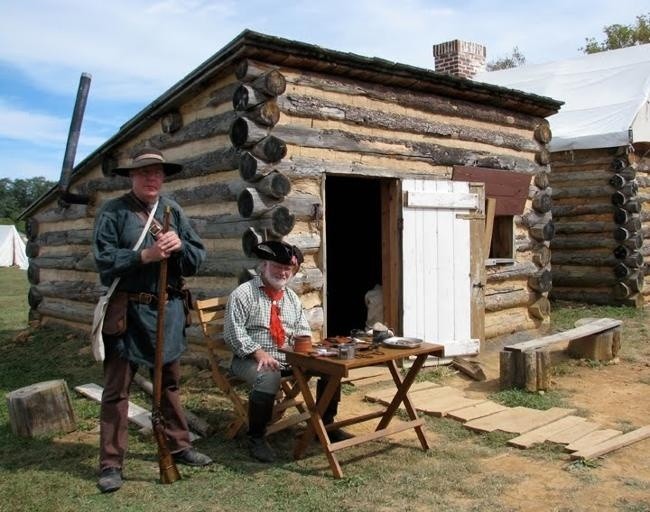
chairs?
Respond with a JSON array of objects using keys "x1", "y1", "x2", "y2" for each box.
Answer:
[{"x1": 195, "y1": 296, "x2": 313, "y2": 458}]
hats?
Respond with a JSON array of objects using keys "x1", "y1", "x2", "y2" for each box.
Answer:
[
  {"x1": 250, "y1": 239, "x2": 304, "y2": 266},
  {"x1": 111, "y1": 148, "x2": 184, "y2": 178}
]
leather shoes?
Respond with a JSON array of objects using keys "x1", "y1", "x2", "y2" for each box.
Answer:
[
  {"x1": 172, "y1": 446, "x2": 213, "y2": 467},
  {"x1": 96, "y1": 466, "x2": 125, "y2": 492}
]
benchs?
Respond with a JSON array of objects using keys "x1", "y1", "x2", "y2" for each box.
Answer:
[{"x1": 499, "y1": 318, "x2": 623, "y2": 394}]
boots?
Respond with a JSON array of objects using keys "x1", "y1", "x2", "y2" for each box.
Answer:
[
  {"x1": 248, "y1": 388, "x2": 278, "y2": 462},
  {"x1": 313, "y1": 378, "x2": 356, "y2": 443}
]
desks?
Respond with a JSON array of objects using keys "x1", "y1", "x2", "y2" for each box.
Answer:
[{"x1": 277, "y1": 337, "x2": 444, "y2": 479}]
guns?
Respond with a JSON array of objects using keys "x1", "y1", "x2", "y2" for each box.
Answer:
[{"x1": 150, "y1": 205, "x2": 182, "y2": 483}]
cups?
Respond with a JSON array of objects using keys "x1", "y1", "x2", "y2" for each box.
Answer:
[{"x1": 294, "y1": 335, "x2": 312, "y2": 352}]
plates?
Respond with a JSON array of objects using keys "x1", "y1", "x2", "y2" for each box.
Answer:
[{"x1": 382, "y1": 337, "x2": 423, "y2": 350}]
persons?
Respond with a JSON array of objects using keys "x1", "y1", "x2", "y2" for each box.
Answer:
[
  {"x1": 224, "y1": 240, "x2": 356, "y2": 464},
  {"x1": 92, "y1": 148, "x2": 213, "y2": 494}
]
